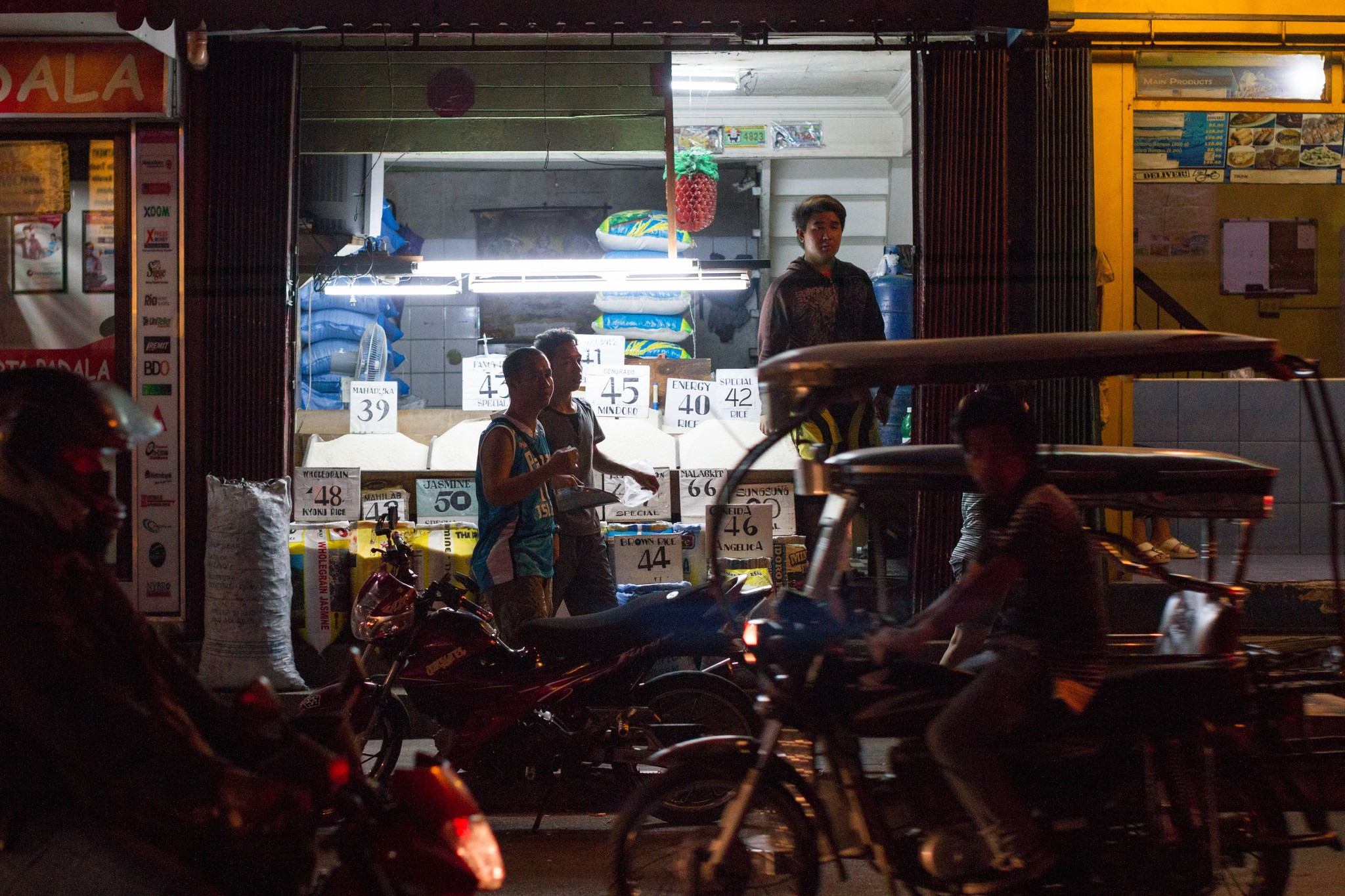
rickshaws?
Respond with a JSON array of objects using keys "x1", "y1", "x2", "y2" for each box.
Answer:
[{"x1": 608, "y1": 330, "x2": 1344, "y2": 896}]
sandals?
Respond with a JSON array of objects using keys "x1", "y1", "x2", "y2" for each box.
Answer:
[
  {"x1": 1131, "y1": 541, "x2": 1170, "y2": 563},
  {"x1": 1151, "y1": 536, "x2": 1197, "y2": 558}
]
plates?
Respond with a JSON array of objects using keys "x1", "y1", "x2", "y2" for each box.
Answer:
[{"x1": 1226, "y1": 111, "x2": 1344, "y2": 169}]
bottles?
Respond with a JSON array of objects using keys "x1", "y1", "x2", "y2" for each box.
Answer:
[{"x1": 901, "y1": 407, "x2": 912, "y2": 445}]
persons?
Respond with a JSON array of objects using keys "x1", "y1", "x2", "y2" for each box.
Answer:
[
  {"x1": 533, "y1": 332, "x2": 659, "y2": 618},
  {"x1": 757, "y1": 194, "x2": 897, "y2": 618},
  {"x1": 82, "y1": 242, "x2": 107, "y2": 291},
  {"x1": 13, "y1": 225, "x2": 58, "y2": 262},
  {"x1": 469, "y1": 347, "x2": 579, "y2": 645},
  {"x1": 0, "y1": 368, "x2": 358, "y2": 896},
  {"x1": 859, "y1": 384, "x2": 1105, "y2": 894}
]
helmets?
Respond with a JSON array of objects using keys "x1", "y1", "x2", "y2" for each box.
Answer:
[{"x1": 1, "y1": 367, "x2": 123, "y2": 450}]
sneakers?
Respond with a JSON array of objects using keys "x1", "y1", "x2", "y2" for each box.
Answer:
[{"x1": 962, "y1": 846, "x2": 1057, "y2": 893}]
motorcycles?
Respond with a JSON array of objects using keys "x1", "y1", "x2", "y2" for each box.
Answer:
[
  {"x1": 324, "y1": 503, "x2": 762, "y2": 835},
  {"x1": 217, "y1": 645, "x2": 506, "y2": 896}
]
